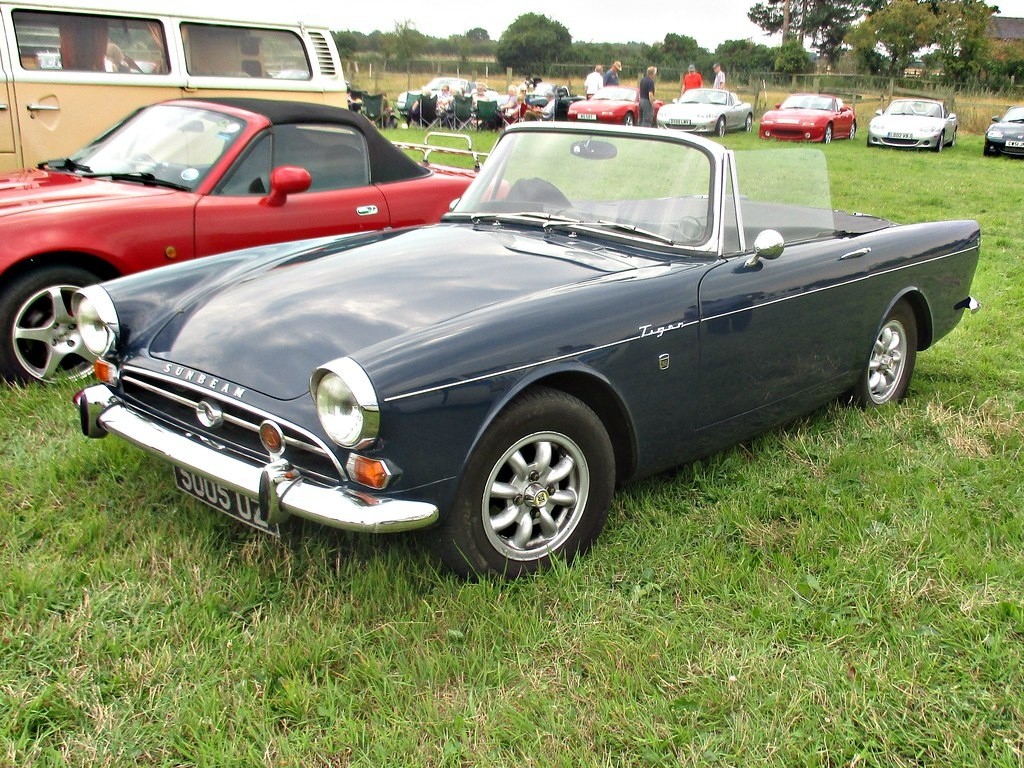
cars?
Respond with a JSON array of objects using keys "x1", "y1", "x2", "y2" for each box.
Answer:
[
  {"x1": 74, "y1": 123, "x2": 983, "y2": 589},
  {"x1": 523, "y1": 85, "x2": 587, "y2": 121},
  {"x1": 395, "y1": 75, "x2": 489, "y2": 120}
]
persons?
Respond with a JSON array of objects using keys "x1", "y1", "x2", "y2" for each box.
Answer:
[
  {"x1": 639, "y1": 66, "x2": 664, "y2": 128},
  {"x1": 500, "y1": 77, "x2": 556, "y2": 123},
  {"x1": 471, "y1": 84, "x2": 489, "y2": 127},
  {"x1": 680, "y1": 64, "x2": 704, "y2": 101},
  {"x1": 584, "y1": 64, "x2": 604, "y2": 101},
  {"x1": 381, "y1": 92, "x2": 400, "y2": 127},
  {"x1": 603, "y1": 61, "x2": 623, "y2": 87},
  {"x1": 345, "y1": 88, "x2": 366, "y2": 113},
  {"x1": 435, "y1": 85, "x2": 463, "y2": 119},
  {"x1": 712, "y1": 62, "x2": 726, "y2": 91},
  {"x1": 401, "y1": 88, "x2": 431, "y2": 130}
]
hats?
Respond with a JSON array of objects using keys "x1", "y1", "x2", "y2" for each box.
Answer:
[
  {"x1": 613, "y1": 60, "x2": 622, "y2": 70},
  {"x1": 711, "y1": 63, "x2": 720, "y2": 69},
  {"x1": 688, "y1": 65, "x2": 695, "y2": 72},
  {"x1": 520, "y1": 87, "x2": 527, "y2": 91}
]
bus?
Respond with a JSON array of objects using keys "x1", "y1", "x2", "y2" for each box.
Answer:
[{"x1": 1, "y1": 1, "x2": 353, "y2": 176}]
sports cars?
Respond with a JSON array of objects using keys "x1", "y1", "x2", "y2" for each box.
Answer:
[
  {"x1": 865, "y1": 99, "x2": 959, "y2": 154},
  {"x1": 0, "y1": 95, "x2": 510, "y2": 388},
  {"x1": 759, "y1": 93, "x2": 857, "y2": 143},
  {"x1": 981, "y1": 106, "x2": 1024, "y2": 161},
  {"x1": 568, "y1": 84, "x2": 666, "y2": 126},
  {"x1": 652, "y1": 89, "x2": 756, "y2": 136}
]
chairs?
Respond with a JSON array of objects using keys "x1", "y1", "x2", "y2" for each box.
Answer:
[
  {"x1": 409, "y1": 93, "x2": 441, "y2": 132},
  {"x1": 360, "y1": 93, "x2": 391, "y2": 130},
  {"x1": 394, "y1": 92, "x2": 422, "y2": 126},
  {"x1": 471, "y1": 99, "x2": 499, "y2": 133},
  {"x1": 443, "y1": 94, "x2": 475, "y2": 132},
  {"x1": 499, "y1": 102, "x2": 527, "y2": 129}
]
